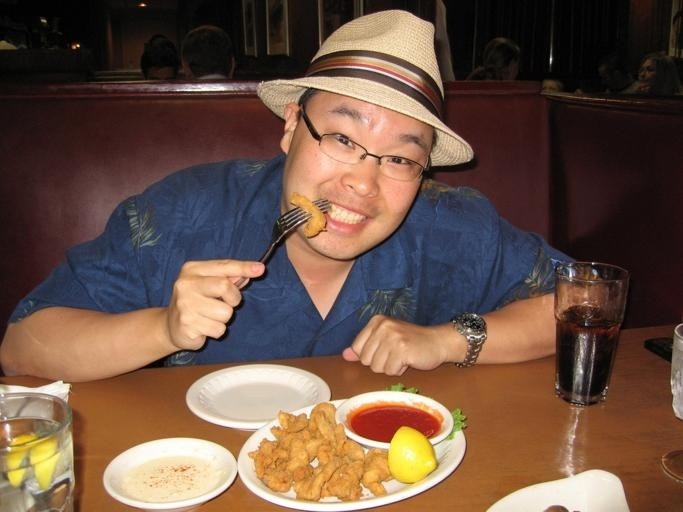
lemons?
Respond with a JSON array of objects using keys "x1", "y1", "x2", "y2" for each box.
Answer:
[
  {"x1": 30, "y1": 436, "x2": 58, "y2": 491},
  {"x1": 6, "y1": 433, "x2": 39, "y2": 488},
  {"x1": 388, "y1": 425, "x2": 438, "y2": 485}
]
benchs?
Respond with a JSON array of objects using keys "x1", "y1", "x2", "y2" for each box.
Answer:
[{"x1": 2, "y1": 81, "x2": 548, "y2": 324}]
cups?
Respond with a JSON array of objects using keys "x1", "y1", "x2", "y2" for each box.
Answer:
[
  {"x1": 0, "y1": 392, "x2": 76, "y2": 512},
  {"x1": 551, "y1": 259, "x2": 630, "y2": 407}
]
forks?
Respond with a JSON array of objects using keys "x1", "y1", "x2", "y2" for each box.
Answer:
[{"x1": 234, "y1": 197, "x2": 336, "y2": 296}]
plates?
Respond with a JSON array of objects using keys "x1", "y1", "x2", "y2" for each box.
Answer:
[
  {"x1": 237, "y1": 390, "x2": 466, "y2": 511},
  {"x1": 182, "y1": 360, "x2": 333, "y2": 433},
  {"x1": 100, "y1": 434, "x2": 238, "y2": 510}
]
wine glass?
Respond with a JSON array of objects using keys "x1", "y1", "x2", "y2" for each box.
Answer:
[{"x1": 663, "y1": 324, "x2": 681, "y2": 480}]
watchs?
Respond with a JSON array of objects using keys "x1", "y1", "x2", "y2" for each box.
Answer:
[{"x1": 450, "y1": 313, "x2": 486, "y2": 368}]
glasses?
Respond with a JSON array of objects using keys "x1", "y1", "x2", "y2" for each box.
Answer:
[{"x1": 299, "y1": 104, "x2": 431, "y2": 183}]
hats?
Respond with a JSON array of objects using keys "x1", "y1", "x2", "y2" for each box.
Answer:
[{"x1": 255, "y1": 11, "x2": 474, "y2": 167}]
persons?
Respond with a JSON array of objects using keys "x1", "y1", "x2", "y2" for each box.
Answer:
[
  {"x1": 0, "y1": 9, "x2": 616, "y2": 383},
  {"x1": 542, "y1": 80, "x2": 563, "y2": 92},
  {"x1": 633, "y1": 53, "x2": 683, "y2": 95},
  {"x1": 466, "y1": 37, "x2": 522, "y2": 80},
  {"x1": 140, "y1": 34, "x2": 178, "y2": 81},
  {"x1": 181, "y1": 25, "x2": 236, "y2": 79}
]
